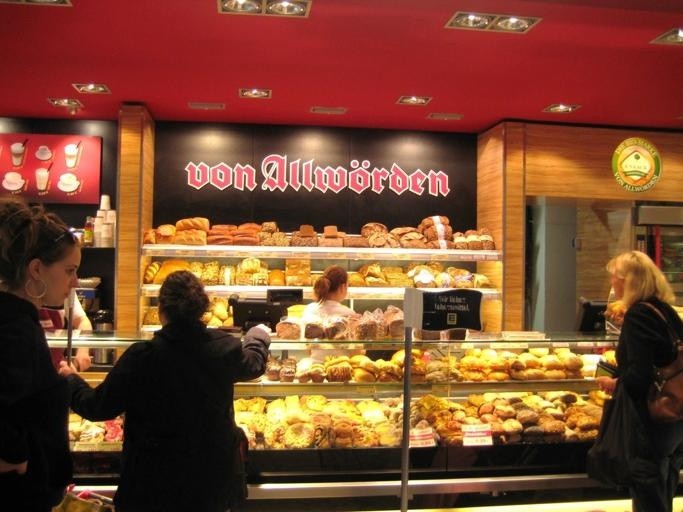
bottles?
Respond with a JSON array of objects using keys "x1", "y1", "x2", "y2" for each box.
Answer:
[{"x1": 84, "y1": 217, "x2": 93, "y2": 248}]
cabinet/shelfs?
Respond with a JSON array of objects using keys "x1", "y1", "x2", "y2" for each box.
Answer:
[
  {"x1": 37, "y1": 328, "x2": 683, "y2": 512},
  {"x1": 139, "y1": 241, "x2": 505, "y2": 361}
]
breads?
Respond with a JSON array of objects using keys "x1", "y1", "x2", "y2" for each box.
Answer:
[
  {"x1": 275, "y1": 307, "x2": 406, "y2": 340},
  {"x1": 233, "y1": 350, "x2": 405, "y2": 449},
  {"x1": 145, "y1": 215, "x2": 496, "y2": 250},
  {"x1": 410, "y1": 350, "x2": 616, "y2": 446},
  {"x1": 199, "y1": 296, "x2": 233, "y2": 328},
  {"x1": 144, "y1": 258, "x2": 492, "y2": 289},
  {"x1": 68, "y1": 413, "x2": 124, "y2": 443},
  {"x1": 142, "y1": 308, "x2": 160, "y2": 325}
]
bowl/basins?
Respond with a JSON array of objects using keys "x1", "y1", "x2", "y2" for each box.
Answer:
[{"x1": 75, "y1": 288, "x2": 101, "y2": 313}]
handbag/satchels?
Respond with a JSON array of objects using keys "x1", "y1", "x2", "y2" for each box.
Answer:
[
  {"x1": 636, "y1": 300, "x2": 683, "y2": 430},
  {"x1": 584, "y1": 381, "x2": 651, "y2": 491},
  {"x1": 232, "y1": 425, "x2": 248, "y2": 512}
]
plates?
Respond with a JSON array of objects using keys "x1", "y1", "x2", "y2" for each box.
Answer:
[
  {"x1": 57, "y1": 181, "x2": 80, "y2": 193},
  {"x1": 34, "y1": 151, "x2": 53, "y2": 161},
  {"x1": 1, "y1": 178, "x2": 24, "y2": 191}
]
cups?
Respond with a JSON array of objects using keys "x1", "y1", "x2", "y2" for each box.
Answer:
[
  {"x1": 39, "y1": 145, "x2": 48, "y2": 156},
  {"x1": 5, "y1": 172, "x2": 22, "y2": 187},
  {"x1": 92, "y1": 193, "x2": 116, "y2": 248},
  {"x1": 65, "y1": 144, "x2": 79, "y2": 169},
  {"x1": 35, "y1": 169, "x2": 50, "y2": 191},
  {"x1": 60, "y1": 173, "x2": 77, "y2": 188},
  {"x1": 10, "y1": 143, "x2": 25, "y2": 166}
]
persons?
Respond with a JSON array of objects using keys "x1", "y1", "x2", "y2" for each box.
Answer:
[
  {"x1": 37, "y1": 287, "x2": 93, "y2": 373},
  {"x1": 596, "y1": 250, "x2": 683, "y2": 512},
  {"x1": 0, "y1": 197, "x2": 82, "y2": 512},
  {"x1": 303, "y1": 264, "x2": 356, "y2": 358},
  {"x1": 57, "y1": 270, "x2": 272, "y2": 511}
]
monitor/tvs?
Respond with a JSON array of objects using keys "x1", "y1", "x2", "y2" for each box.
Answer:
[
  {"x1": 233, "y1": 299, "x2": 288, "y2": 333},
  {"x1": 574, "y1": 301, "x2": 613, "y2": 333}
]
